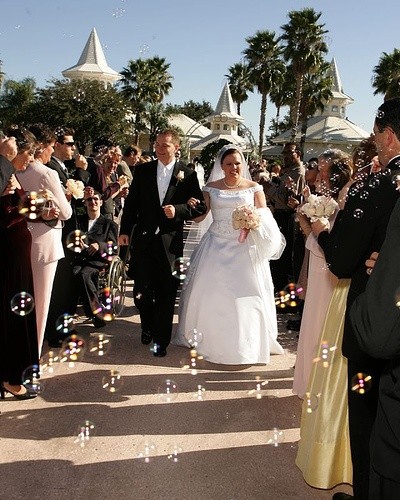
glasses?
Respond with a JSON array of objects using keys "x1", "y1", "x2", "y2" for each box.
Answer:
[
  {"x1": 64, "y1": 142, "x2": 74, "y2": 146},
  {"x1": 86, "y1": 197, "x2": 98, "y2": 201},
  {"x1": 280, "y1": 149, "x2": 296, "y2": 154}
]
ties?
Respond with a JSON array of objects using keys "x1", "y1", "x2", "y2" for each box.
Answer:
[{"x1": 158, "y1": 166, "x2": 168, "y2": 204}]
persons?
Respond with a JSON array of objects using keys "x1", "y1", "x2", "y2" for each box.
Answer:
[
  {"x1": 0, "y1": 123, "x2": 154, "y2": 399},
  {"x1": 175, "y1": 144, "x2": 285, "y2": 364},
  {"x1": 251, "y1": 97, "x2": 400, "y2": 500},
  {"x1": 119, "y1": 129, "x2": 208, "y2": 357}
]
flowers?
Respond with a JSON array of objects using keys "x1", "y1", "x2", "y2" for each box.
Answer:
[
  {"x1": 66, "y1": 178, "x2": 85, "y2": 200},
  {"x1": 175, "y1": 171, "x2": 185, "y2": 187},
  {"x1": 249, "y1": 150, "x2": 260, "y2": 162},
  {"x1": 233, "y1": 204, "x2": 258, "y2": 242},
  {"x1": 38, "y1": 188, "x2": 53, "y2": 202},
  {"x1": 301, "y1": 194, "x2": 338, "y2": 221}
]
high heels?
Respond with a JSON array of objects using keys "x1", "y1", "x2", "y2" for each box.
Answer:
[{"x1": 1, "y1": 381, "x2": 37, "y2": 400}]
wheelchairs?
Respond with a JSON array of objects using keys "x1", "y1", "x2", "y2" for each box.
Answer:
[{"x1": 78, "y1": 245, "x2": 131, "y2": 329}]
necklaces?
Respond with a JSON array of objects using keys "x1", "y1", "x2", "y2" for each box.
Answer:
[{"x1": 223, "y1": 178, "x2": 242, "y2": 189}]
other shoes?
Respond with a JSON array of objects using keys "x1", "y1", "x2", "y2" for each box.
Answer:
[
  {"x1": 332, "y1": 492, "x2": 354, "y2": 500},
  {"x1": 156, "y1": 345, "x2": 167, "y2": 356},
  {"x1": 286, "y1": 319, "x2": 300, "y2": 331},
  {"x1": 142, "y1": 328, "x2": 152, "y2": 345}
]
